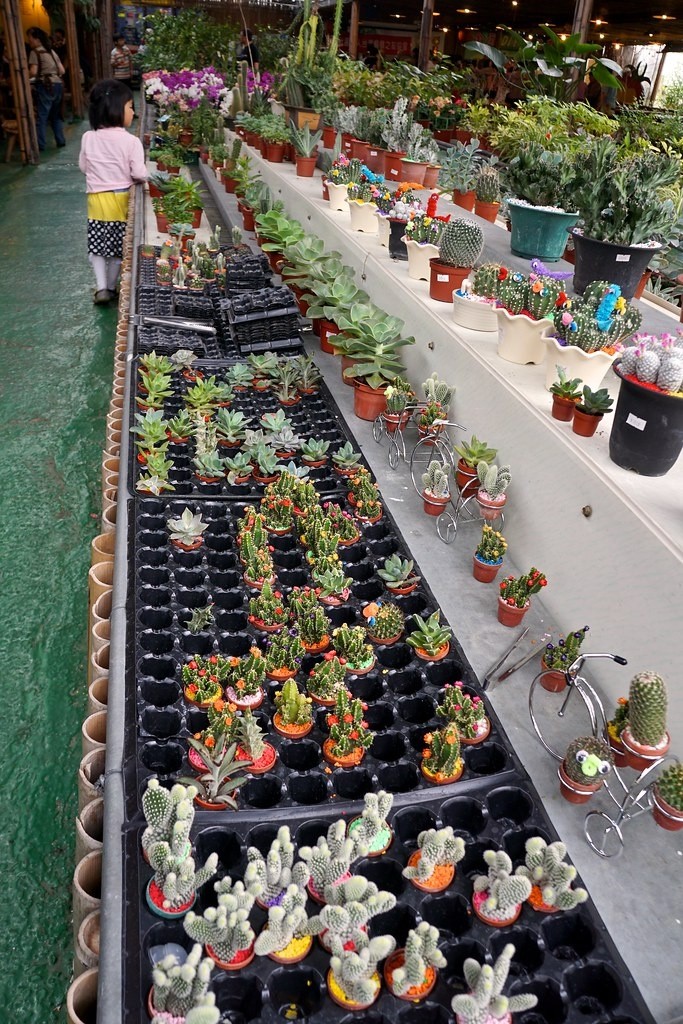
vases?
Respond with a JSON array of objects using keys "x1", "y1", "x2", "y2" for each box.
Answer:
[
  {"x1": 266, "y1": 668, "x2": 300, "y2": 681},
  {"x1": 324, "y1": 178, "x2": 350, "y2": 211},
  {"x1": 343, "y1": 197, "x2": 379, "y2": 233},
  {"x1": 471, "y1": 548, "x2": 501, "y2": 584},
  {"x1": 188, "y1": 743, "x2": 227, "y2": 770},
  {"x1": 609, "y1": 356, "x2": 683, "y2": 476},
  {"x1": 417, "y1": 424, "x2": 445, "y2": 447},
  {"x1": 225, "y1": 684, "x2": 263, "y2": 711},
  {"x1": 356, "y1": 508, "x2": 383, "y2": 525},
  {"x1": 461, "y1": 715, "x2": 490, "y2": 744},
  {"x1": 400, "y1": 235, "x2": 440, "y2": 281},
  {"x1": 496, "y1": 595, "x2": 530, "y2": 628},
  {"x1": 490, "y1": 302, "x2": 551, "y2": 366},
  {"x1": 420, "y1": 755, "x2": 463, "y2": 786},
  {"x1": 540, "y1": 653, "x2": 575, "y2": 693},
  {"x1": 266, "y1": 521, "x2": 294, "y2": 536},
  {"x1": 368, "y1": 632, "x2": 402, "y2": 645},
  {"x1": 324, "y1": 735, "x2": 367, "y2": 769},
  {"x1": 452, "y1": 290, "x2": 500, "y2": 332},
  {"x1": 302, "y1": 633, "x2": 332, "y2": 653},
  {"x1": 338, "y1": 525, "x2": 361, "y2": 545},
  {"x1": 185, "y1": 683, "x2": 222, "y2": 708},
  {"x1": 372, "y1": 209, "x2": 389, "y2": 246},
  {"x1": 251, "y1": 621, "x2": 285, "y2": 632},
  {"x1": 309, "y1": 686, "x2": 354, "y2": 706},
  {"x1": 541, "y1": 325, "x2": 621, "y2": 391}
]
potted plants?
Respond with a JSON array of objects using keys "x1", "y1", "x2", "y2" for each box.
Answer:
[
  {"x1": 131, "y1": 53, "x2": 683, "y2": 1024},
  {"x1": 428, "y1": 216, "x2": 487, "y2": 303}
]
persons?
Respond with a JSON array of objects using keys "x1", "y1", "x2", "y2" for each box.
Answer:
[
  {"x1": 77, "y1": 79, "x2": 146, "y2": 304},
  {"x1": 26, "y1": 27, "x2": 65, "y2": 153},
  {"x1": 333, "y1": 40, "x2": 602, "y2": 109},
  {"x1": 109, "y1": 32, "x2": 138, "y2": 120},
  {"x1": 239, "y1": 28, "x2": 259, "y2": 66},
  {"x1": 48, "y1": 29, "x2": 67, "y2": 62}
]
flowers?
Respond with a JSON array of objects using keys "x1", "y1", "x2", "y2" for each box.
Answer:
[
  {"x1": 329, "y1": 152, "x2": 361, "y2": 183},
  {"x1": 261, "y1": 491, "x2": 293, "y2": 528},
  {"x1": 612, "y1": 327, "x2": 683, "y2": 392},
  {"x1": 499, "y1": 565, "x2": 550, "y2": 607},
  {"x1": 544, "y1": 622, "x2": 590, "y2": 670},
  {"x1": 347, "y1": 179, "x2": 382, "y2": 204},
  {"x1": 140, "y1": 61, "x2": 227, "y2": 116},
  {"x1": 307, "y1": 649, "x2": 349, "y2": 696},
  {"x1": 450, "y1": 261, "x2": 513, "y2": 300},
  {"x1": 228, "y1": 645, "x2": 267, "y2": 697},
  {"x1": 295, "y1": 589, "x2": 330, "y2": 641},
  {"x1": 476, "y1": 522, "x2": 508, "y2": 561},
  {"x1": 493, "y1": 254, "x2": 577, "y2": 316},
  {"x1": 419, "y1": 400, "x2": 443, "y2": 425},
  {"x1": 244, "y1": 68, "x2": 283, "y2": 98},
  {"x1": 192, "y1": 702, "x2": 241, "y2": 751},
  {"x1": 540, "y1": 277, "x2": 643, "y2": 351},
  {"x1": 435, "y1": 681, "x2": 485, "y2": 737},
  {"x1": 184, "y1": 650, "x2": 229, "y2": 701},
  {"x1": 355, "y1": 479, "x2": 382, "y2": 516},
  {"x1": 404, "y1": 213, "x2": 446, "y2": 243},
  {"x1": 323, "y1": 690, "x2": 377, "y2": 752},
  {"x1": 248, "y1": 580, "x2": 290, "y2": 624},
  {"x1": 419, "y1": 720, "x2": 465, "y2": 782},
  {"x1": 367, "y1": 597, "x2": 404, "y2": 635},
  {"x1": 265, "y1": 623, "x2": 304, "y2": 671},
  {"x1": 374, "y1": 185, "x2": 419, "y2": 214},
  {"x1": 323, "y1": 501, "x2": 359, "y2": 539}
]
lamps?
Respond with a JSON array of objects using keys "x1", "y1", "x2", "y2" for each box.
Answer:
[
  {"x1": 589, "y1": 18, "x2": 611, "y2": 24},
  {"x1": 611, "y1": 41, "x2": 624, "y2": 50},
  {"x1": 418, "y1": 9, "x2": 441, "y2": 17},
  {"x1": 495, "y1": 25, "x2": 513, "y2": 30},
  {"x1": 389, "y1": 12, "x2": 407, "y2": 19},
  {"x1": 537, "y1": 22, "x2": 557, "y2": 28},
  {"x1": 652, "y1": 13, "x2": 676, "y2": 20},
  {"x1": 463, "y1": 26, "x2": 480, "y2": 31},
  {"x1": 456, "y1": 7, "x2": 479, "y2": 15},
  {"x1": 593, "y1": 32, "x2": 611, "y2": 39},
  {"x1": 557, "y1": 32, "x2": 571, "y2": 40}
]
